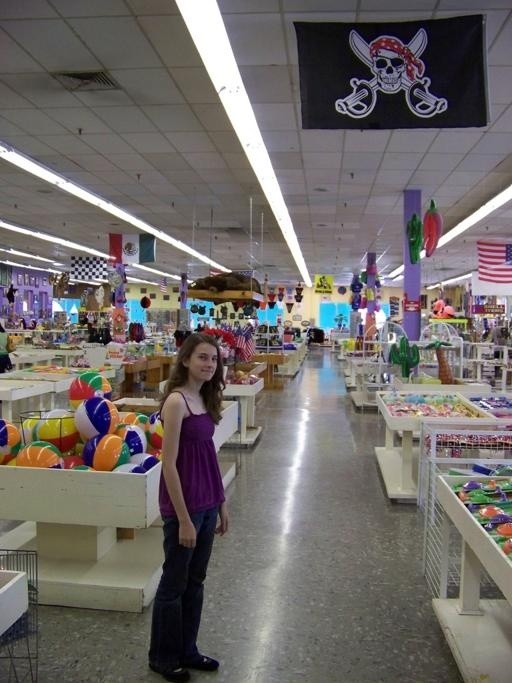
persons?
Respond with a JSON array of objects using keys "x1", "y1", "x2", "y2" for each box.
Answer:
[{"x1": 148, "y1": 331, "x2": 230, "y2": 682}]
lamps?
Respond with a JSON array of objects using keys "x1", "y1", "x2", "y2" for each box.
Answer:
[
  {"x1": 388, "y1": 264, "x2": 404, "y2": 282},
  {"x1": 0, "y1": 143, "x2": 232, "y2": 287},
  {"x1": 420, "y1": 186, "x2": 511, "y2": 260},
  {"x1": 177, "y1": 1, "x2": 315, "y2": 286}
]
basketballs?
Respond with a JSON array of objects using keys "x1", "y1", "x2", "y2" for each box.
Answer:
[
  {"x1": 0, "y1": 398, "x2": 163, "y2": 473},
  {"x1": 68, "y1": 370, "x2": 114, "y2": 411}
]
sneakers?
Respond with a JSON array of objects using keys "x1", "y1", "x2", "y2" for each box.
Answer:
[{"x1": 149, "y1": 653, "x2": 219, "y2": 683}]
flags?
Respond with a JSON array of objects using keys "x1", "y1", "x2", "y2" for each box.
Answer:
[
  {"x1": 68, "y1": 254, "x2": 117, "y2": 281},
  {"x1": 109, "y1": 230, "x2": 158, "y2": 264},
  {"x1": 476, "y1": 239, "x2": 512, "y2": 286},
  {"x1": 174, "y1": 319, "x2": 256, "y2": 363},
  {"x1": 291, "y1": 13, "x2": 487, "y2": 128},
  {"x1": 159, "y1": 279, "x2": 169, "y2": 293}
]
